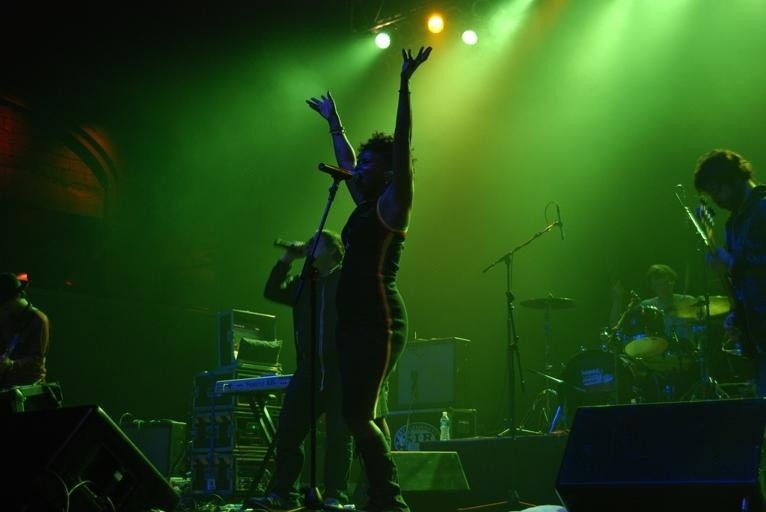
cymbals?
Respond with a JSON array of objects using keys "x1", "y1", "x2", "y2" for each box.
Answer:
[
  {"x1": 677, "y1": 295, "x2": 730, "y2": 319},
  {"x1": 520, "y1": 298, "x2": 577, "y2": 309}
]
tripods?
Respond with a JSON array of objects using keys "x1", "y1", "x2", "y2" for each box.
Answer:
[
  {"x1": 518, "y1": 311, "x2": 570, "y2": 433},
  {"x1": 277, "y1": 276, "x2": 354, "y2": 512},
  {"x1": 677, "y1": 324, "x2": 729, "y2": 402},
  {"x1": 493, "y1": 266, "x2": 543, "y2": 441}
]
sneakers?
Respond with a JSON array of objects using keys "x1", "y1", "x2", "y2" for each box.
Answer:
[
  {"x1": 322, "y1": 497, "x2": 343, "y2": 510},
  {"x1": 247, "y1": 495, "x2": 308, "y2": 512}
]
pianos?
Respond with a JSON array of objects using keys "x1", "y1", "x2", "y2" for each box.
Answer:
[{"x1": 213, "y1": 374, "x2": 296, "y2": 394}]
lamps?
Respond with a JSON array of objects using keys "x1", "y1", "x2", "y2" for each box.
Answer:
[{"x1": 369, "y1": 0, "x2": 481, "y2": 51}]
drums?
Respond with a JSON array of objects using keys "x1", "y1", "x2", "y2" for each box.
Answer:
[
  {"x1": 663, "y1": 336, "x2": 696, "y2": 373},
  {"x1": 561, "y1": 344, "x2": 659, "y2": 407},
  {"x1": 622, "y1": 306, "x2": 668, "y2": 336}
]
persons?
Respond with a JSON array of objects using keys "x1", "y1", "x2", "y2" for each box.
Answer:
[
  {"x1": 306, "y1": 46, "x2": 434, "y2": 511},
  {"x1": 0, "y1": 266, "x2": 49, "y2": 388},
  {"x1": 694, "y1": 148, "x2": 765, "y2": 397},
  {"x1": 248, "y1": 229, "x2": 355, "y2": 509},
  {"x1": 626, "y1": 264, "x2": 695, "y2": 313}
]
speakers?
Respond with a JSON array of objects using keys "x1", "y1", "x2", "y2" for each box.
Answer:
[
  {"x1": 555, "y1": 397, "x2": 765, "y2": 512},
  {"x1": 0, "y1": 406, "x2": 180, "y2": 512},
  {"x1": 353, "y1": 451, "x2": 470, "y2": 512}
]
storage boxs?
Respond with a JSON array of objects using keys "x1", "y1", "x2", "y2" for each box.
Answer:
[
  {"x1": 385, "y1": 406, "x2": 479, "y2": 451},
  {"x1": 190, "y1": 310, "x2": 288, "y2": 493}
]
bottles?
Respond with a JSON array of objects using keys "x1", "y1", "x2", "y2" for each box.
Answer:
[{"x1": 439, "y1": 411, "x2": 450, "y2": 440}]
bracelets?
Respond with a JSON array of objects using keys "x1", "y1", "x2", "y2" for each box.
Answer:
[
  {"x1": 397, "y1": 89, "x2": 410, "y2": 94},
  {"x1": 329, "y1": 127, "x2": 346, "y2": 135}
]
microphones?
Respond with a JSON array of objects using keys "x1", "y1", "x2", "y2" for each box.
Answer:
[
  {"x1": 319, "y1": 163, "x2": 362, "y2": 183},
  {"x1": 275, "y1": 239, "x2": 309, "y2": 257},
  {"x1": 557, "y1": 207, "x2": 565, "y2": 240}
]
